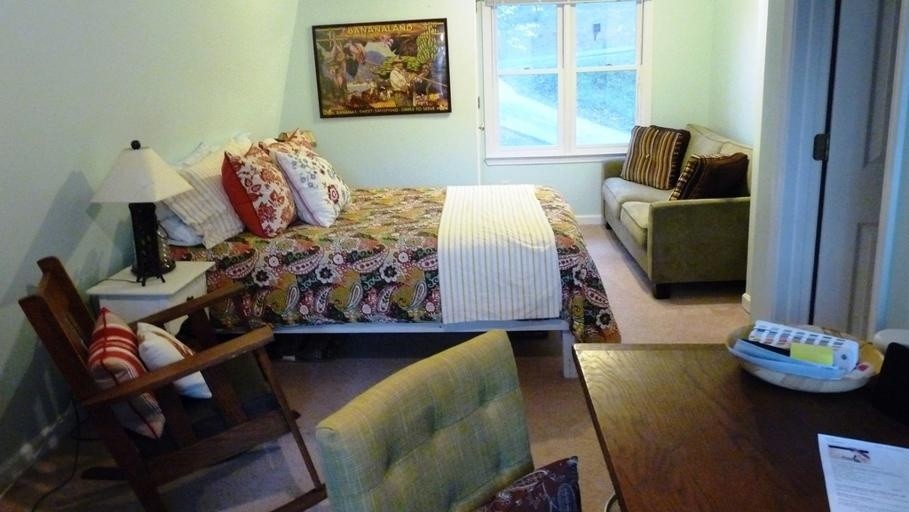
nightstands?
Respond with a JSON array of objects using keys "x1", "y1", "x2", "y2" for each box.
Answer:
[{"x1": 84, "y1": 262, "x2": 212, "y2": 338}]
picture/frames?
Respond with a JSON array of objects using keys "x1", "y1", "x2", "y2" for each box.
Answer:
[{"x1": 312, "y1": 18, "x2": 452, "y2": 118}]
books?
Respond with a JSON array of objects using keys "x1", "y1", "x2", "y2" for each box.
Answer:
[{"x1": 733, "y1": 339, "x2": 821, "y2": 368}]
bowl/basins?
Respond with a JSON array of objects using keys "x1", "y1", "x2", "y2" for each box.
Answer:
[{"x1": 725, "y1": 320, "x2": 886, "y2": 394}]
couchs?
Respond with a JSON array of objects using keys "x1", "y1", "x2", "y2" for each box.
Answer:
[{"x1": 601, "y1": 123, "x2": 754, "y2": 300}]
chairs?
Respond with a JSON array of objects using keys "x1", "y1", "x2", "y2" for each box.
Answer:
[
  {"x1": 18, "y1": 255, "x2": 327, "y2": 512},
  {"x1": 315, "y1": 328, "x2": 535, "y2": 512}
]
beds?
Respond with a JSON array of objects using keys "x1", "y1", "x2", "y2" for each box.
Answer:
[{"x1": 134, "y1": 128, "x2": 622, "y2": 379}]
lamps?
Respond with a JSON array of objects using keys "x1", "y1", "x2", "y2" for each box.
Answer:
[{"x1": 89, "y1": 141, "x2": 195, "y2": 286}]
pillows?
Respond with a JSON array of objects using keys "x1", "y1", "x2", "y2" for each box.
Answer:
[
  {"x1": 620, "y1": 125, "x2": 691, "y2": 191},
  {"x1": 88, "y1": 307, "x2": 212, "y2": 439},
  {"x1": 472, "y1": 456, "x2": 581, "y2": 512},
  {"x1": 154, "y1": 129, "x2": 352, "y2": 250},
  {"x1": 667, "y1": 153, "x2": 749, "y2": 200}
]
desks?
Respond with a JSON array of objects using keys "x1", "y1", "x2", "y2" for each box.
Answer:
[{"x1": 571, "y1": 342, "x2": 909, "y2": 512}]
